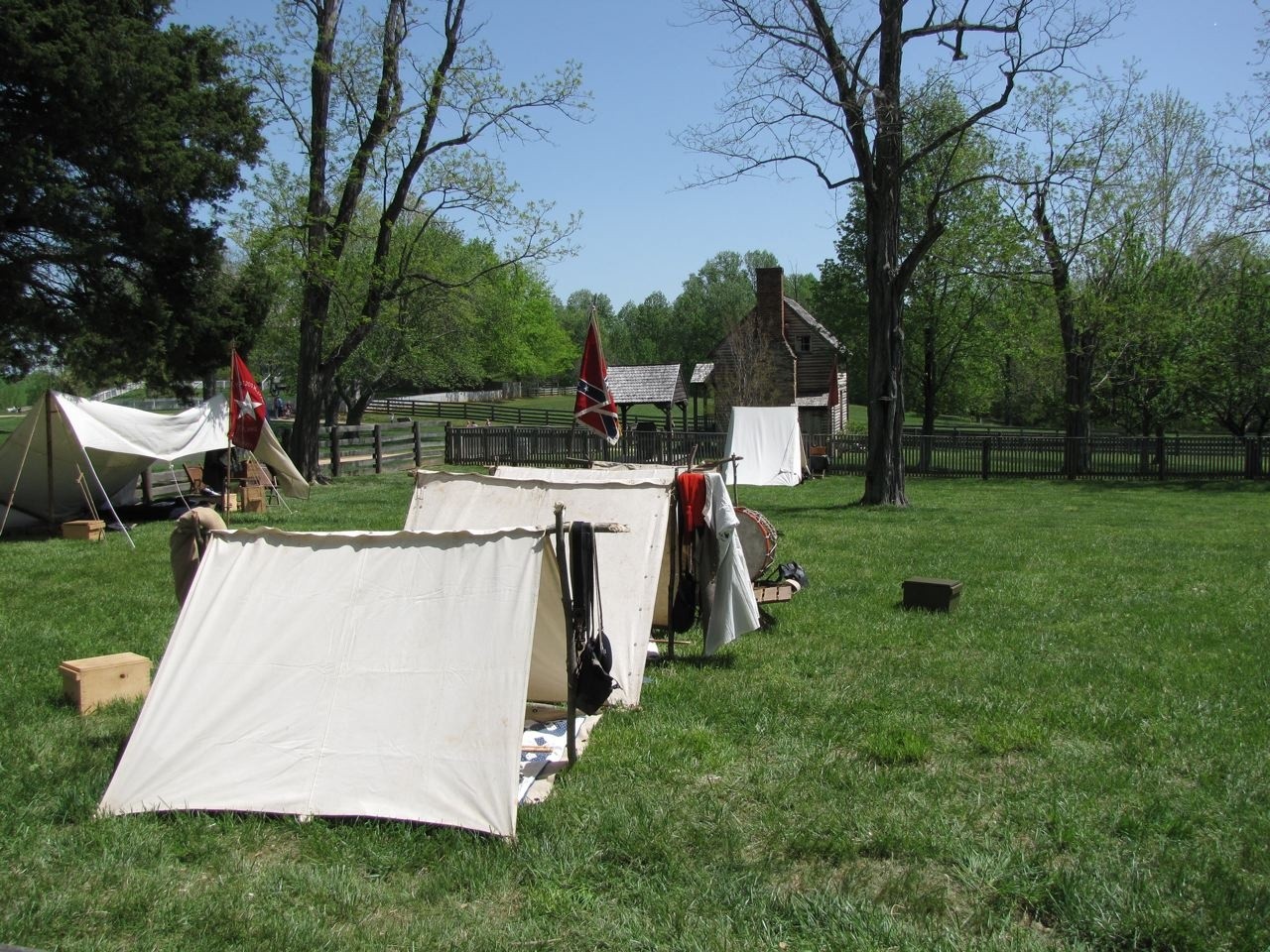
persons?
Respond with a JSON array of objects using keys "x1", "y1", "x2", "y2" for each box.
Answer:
[
  {"x1": 465, "y1": 418, "x2": 491, "y2": 427},
  {"x1": 273, "y1": 395, "x2": 292, "y2": 419}
]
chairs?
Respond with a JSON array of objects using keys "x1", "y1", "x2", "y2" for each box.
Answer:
[
  {"x1": 254, "y1": 463, "x2": 281, "y2": 508},
  {"x1": 183, "y1": 461, "x2": 207, "y2": 493}
]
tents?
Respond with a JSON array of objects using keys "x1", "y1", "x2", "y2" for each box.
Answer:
[
  {"x1": 1, "y1": 373, "x2": 310, "y2": 540},
  {"x1": 91, "y1": 518, "x2": 611, "y2": 845},
  {"x1": 399, "y1": 458, "x2": 747, "y2": 713},
  {"x1": 720, "y1": 406, "x2": 813, "y2": 487}
]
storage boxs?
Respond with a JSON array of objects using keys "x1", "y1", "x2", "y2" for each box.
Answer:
[
  {"x1": 60, "y1": 647, "x2": 151, "y2": 718},
  {"x1": 900, "y1": 576, "x2": 963, "y2": 614},
  {"x1": 60, "y1": 518, "x2": 107, "y2": 540}
]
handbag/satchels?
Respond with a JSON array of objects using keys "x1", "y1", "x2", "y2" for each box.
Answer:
[
  {"x1": 574, "y1": 631, "x2": 613, "y2": 715},
  {"x1": 672, "y1": 572, "x2": 696, "y2": 634}
]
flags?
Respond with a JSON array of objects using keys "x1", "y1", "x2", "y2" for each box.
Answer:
[
  {"x1": 228, "y1": 348, "x2": 266, "y2": 453},
  {"x1": 571, "y1": 305, "x2": 622, "y2": 446}
]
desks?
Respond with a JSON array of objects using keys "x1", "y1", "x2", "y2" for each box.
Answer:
[{"x1": 224, "y1": 477, "x2": 257, "y2": 487}]
